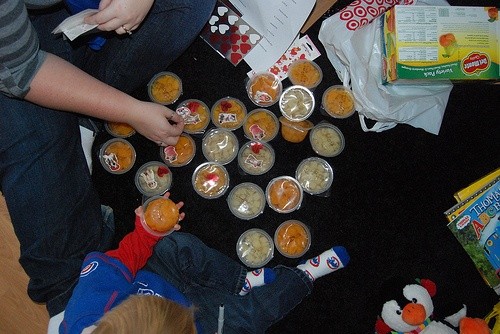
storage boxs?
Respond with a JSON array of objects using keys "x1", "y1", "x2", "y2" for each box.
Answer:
[{"x1": 379, "y1": 4, "x2": 500, "y2": 84}]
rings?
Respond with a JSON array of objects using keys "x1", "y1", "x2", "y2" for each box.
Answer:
[
  {"x1": 170, "y1": 111, "x2": 174, "y2": 120},
  {"x1": 160, "y1": 143, "x2": 162, "y2": 145},
  {"x1": 122, "y1": 25, "x2": 129, "y2": 32}
]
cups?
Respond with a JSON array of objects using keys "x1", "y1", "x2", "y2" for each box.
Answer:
[
  {"x1": 175, "y1": 99, "x2": 209, "y2": 135},
  {"x1": 149, "y1": 72, "x2": 182, "y2": 105},
  {"x1": 140, "y1": 195, "x2": 180, "y2": 236},
  {"x1": 242, "y1": 108, "x2": 279, "y2": 144},
  {"x1": 99, "y1": 137, "x2": 136, "y2": 174},
  {"x1": 227, "y1": 182, "x2": 265, "y2": 219},
  {"x1": 238, "y1": 141, "x2": 276, "y2": 175},
  {"x1": 246, "y1": 70, "x2": 282, "y2": 107},
  {"x1": 211, "y1": 97, "x2": 247, "y2": 132},
  {"x1": 104, "y1": 120, "x2": 136, "y2": 137},
  {"x1": 322, "y1": 85, "x2": 355, "y2": 118},
  {"x1": 134, "y1": 161, "x2": 173, "y2": 197},
  {"x1": 287, "y1": 59, "x2": 323, "y2": 92},
  {"x1": 192, "y1": 161, "x2": 230, "y2": 199},
  {"x1": 160, "y1": 132, "x2": 196, "y2": 167},
  {"x1": 236, "y1": 229, "x2": 274, "y2": 268},
  {"x1": 279, "y1": 85, "x2": 316, "y2": 122},
  {"x1": 266, "y1": 175, "x2": 303, "y2": 214},
  {"x1": 201, "y1": 128, "x2": 238, "y2": 165},
  {"x1": 279, "y1": 115, "x2": 314, "y2": 142},
  {"x1": 295, "y1": 156, "x2": 334, "y2": 195},
  {"x1": 309, "y1": 122, "x2": 344, "y2": 157},
  {"x1": 274, "y1": 220, "x2": 312, "y2": 258}
]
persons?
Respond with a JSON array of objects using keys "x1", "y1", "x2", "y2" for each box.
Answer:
[
  {"x1": 0, "y1": 0, "x2": 216, "y2": 334},
  {"x1": 58, "y1": 193, "x2": 350, "y2": 334}
]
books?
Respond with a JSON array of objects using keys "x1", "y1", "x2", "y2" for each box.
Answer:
[{"x1": 444, "y1": 168, "x2": 500, "y2": 300}]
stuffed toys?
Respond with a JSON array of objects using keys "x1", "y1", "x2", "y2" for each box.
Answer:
[
  {"x1": 458, "y1": 302, "x2": 500, "y2": 334},
  {"x1": 374, "y1": 277, "x2": 467, "y2": 334}
]
